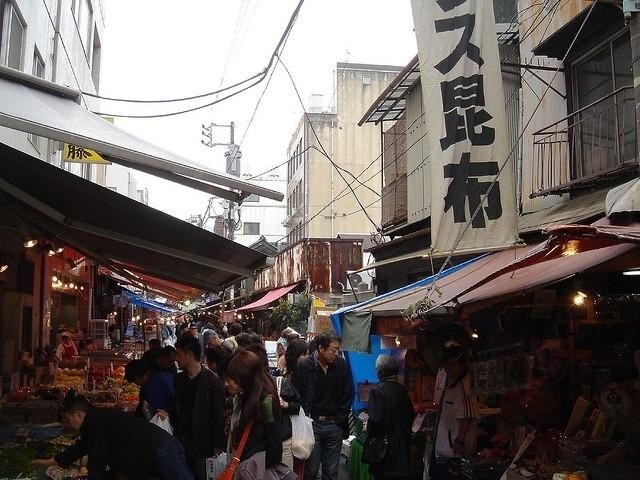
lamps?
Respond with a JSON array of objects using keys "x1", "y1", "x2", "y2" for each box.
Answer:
[
  {"x1": 394, "y1": 336, "x2": 401, "y2": 348},
  {"x1": 572, "y1": 272, "x2": 587, "y2": 307},
  {"x1": 472, "y1": 327, "x2": 480, "y2": 339},
  {"x1": 20, "y1": 231, "x2": 84, "y2": 291},
  {"x1": 0, "y1": 261, "x2": 9, "y2": 273}
]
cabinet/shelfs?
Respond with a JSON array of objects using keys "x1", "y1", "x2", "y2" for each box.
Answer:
[{"x1": 88, "y1": 319, "x2": 109, "y2": 344}]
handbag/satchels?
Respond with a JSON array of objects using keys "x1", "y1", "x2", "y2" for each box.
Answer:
[
  {"x1": 206, "y1": 452, "x2": 227, "y2": 480},
  {"x1": 291, "y1": 414, "x2": 315, "y2": 462},
  {"x1": 361, "y1": 434, "x2": 387, "y2": 463}
]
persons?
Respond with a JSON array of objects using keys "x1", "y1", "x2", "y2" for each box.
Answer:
[
  {"x1": 157, "y1": 334, "x2": 225, "y2": 479},
  {"x1": 362, "y1": 358, "x2": 414, "y2": 479},
  {"x1": 126, "y1": 360, "x2": 175, "y2": 417},
  {"x1": 32, "y1": 396, "x2": 194, "y2": 479},
  {"x1": 280, "y1": 339, "x2": 310, "y2": 480},
  {"x1": 291, "y1": 331, "x2": 356, "y2": 479},
  {"x1": 418, "y1": 341, "x2": 477, "y2": 479},
  {"x1": 56, "y1": 332, "x2": 78, "y2": 360},
  {"x1": 141, "y1": 319, "x2": 300, "y2": 377},
  {"x1": 227, "y1": 350, "x2": 292, "y2": 479}
]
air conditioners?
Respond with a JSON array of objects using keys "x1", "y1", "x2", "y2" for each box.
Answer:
[{"x1": 345, "y1": 269, "x2": 373, "y2": 291}]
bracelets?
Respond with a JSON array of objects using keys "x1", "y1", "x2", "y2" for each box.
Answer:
[{"x1": 455, "y1": 439, "x2": 464, "y2": 443}]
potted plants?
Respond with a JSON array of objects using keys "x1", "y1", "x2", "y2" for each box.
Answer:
[{"x1": 29, "y1": 343, "x2": 57, "y2": 385}]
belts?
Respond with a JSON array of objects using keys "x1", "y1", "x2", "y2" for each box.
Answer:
[{"x1": 311, "y1": 414, "x2": 334, "y2": 421}]
